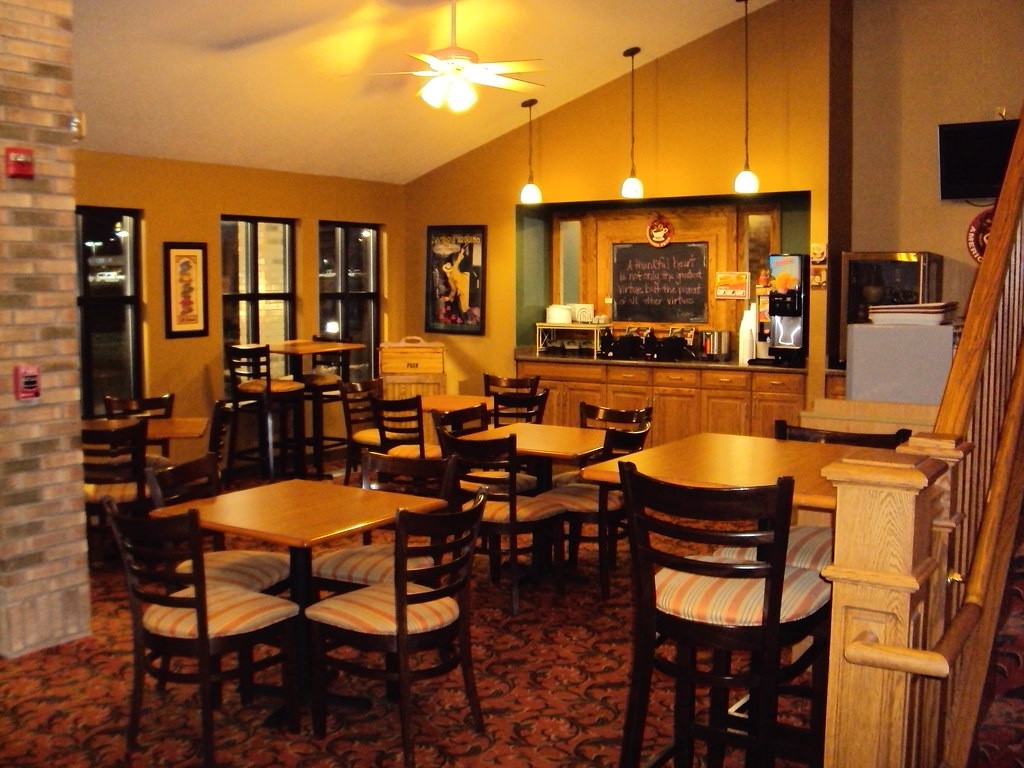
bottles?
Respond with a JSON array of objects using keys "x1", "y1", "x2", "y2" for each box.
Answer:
[
  {"x1": 645, "y1": 330, "x2": 652, "y2": 360},
  {"x1": 602, "y1": 327, "x2": 613, "y2": 358}
]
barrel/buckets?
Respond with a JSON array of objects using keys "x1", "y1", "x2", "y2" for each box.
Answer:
[{"x1": 703, "y1": 330, "x2": 730, "y2": 360}]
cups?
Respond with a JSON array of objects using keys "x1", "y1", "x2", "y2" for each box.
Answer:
[{"x1": 739, "y1": 303, "x2": 757, "y2": 366}]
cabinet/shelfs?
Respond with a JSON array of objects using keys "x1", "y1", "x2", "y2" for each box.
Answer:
[
  {"x1": 517, "y1": 361, "x2": 608, "y2": 475},
  {"x1": 607, "y1": 365, "x2": 702, "y2": 452},
  {"x1": 701, "y1": 369, "x2": 806, "y2": 439}
]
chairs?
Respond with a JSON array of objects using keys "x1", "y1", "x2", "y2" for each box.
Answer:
[
  {"x1": 431, "y1": 402, "x2": 537, "y2": 550},
  {"x1": 144, "y1": 451, "x2": 291, "y2": 710},
  {"x1": 536, "y1": 422, "x2": 653, "y2": 601},
  {"x1": 282, "y1": 335, "x2": 358, "y2": 480},
  {"x1": 311, "y1": 447, "x2": 463, "y2": 663},
  {"x1": 100, "y1": 494, "x2": 302, "y2": 768},
  {"x1": 490, "y1": 388, "x2": 550, "y2": 429},
  {"x1": 337, "y1": 376, "x2": 397, "y2": 485},
  {"x1": 712, "y1": 419, "x2": 913, "y2": 571},
  {"x1": 617, "y1": 459, "x2": 832, "y2": 768},
  {"x1": 436, "y1": 425, "x2": 566, "y2": 618},
  {"x1": 368, "y1": 394, "x2": 442, "y2": 486},
  {"x1": 103, "y1": 393, "x2": 185, "y2": 498},
  {"x1": 303, "y1": 487, "x2": 490, "y2": 768},
  {"x1": 483, "y1": 373, "x2": 540, "y2": 429},
  {"x1": 167, "y1": 399, "x2": 235, "y2": 493},
  {"x1": 81, "y1": 417, "x2": 150, "y2": 573},
  {"x1": 224, "y1": 342, "x2": 305, "y2": 483},
  {"x1": 552, "y1": 400, "x2": 654, "y2": 573}
]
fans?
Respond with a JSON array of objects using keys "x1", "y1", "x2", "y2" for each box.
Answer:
[{"x1": 369, "y1": 0, "x2": 553, "y2": 96}]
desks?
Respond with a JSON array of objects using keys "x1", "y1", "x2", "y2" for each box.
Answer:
[
  {"x1": 535, "y1": 322, "x2": 613, "y2": 360},
  {"x1": 578, "y1": 431, "x2": 895, "y2": 767},
  {"x1": 149, "y1": 479, "x2": 449, "y2": 730},
  {"x1": 81, "y1": 416, "x2": 210, "y2": 567},
  {"x1": 458, "y1": 422, "x2": 607, "y2": 587},
  {"x1": 270, "y1": 339, "x2": 366, "y2": 482},
  {"x1": 420, "y1": 394, "x2": 508, "y2": 431}
]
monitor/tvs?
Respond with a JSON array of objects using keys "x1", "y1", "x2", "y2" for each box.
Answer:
[{"x1": 937, "y1": 118, "x2": 1020, "y2": 201}]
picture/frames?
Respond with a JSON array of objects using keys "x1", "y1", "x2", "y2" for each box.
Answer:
[
  {"x1": 163, "y1": 241, "x2": 209, "y2": 340},
  {"x1": 425, "y1": 224, "x2": 488, "y2": 337}
]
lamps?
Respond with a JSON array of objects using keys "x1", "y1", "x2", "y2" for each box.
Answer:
[
  {"x1": 734, "y1": 0, "x2": 759, "y2": 193},
  {"x1": 621, "y1": 55, "x2": 643, "y2": 199},
  {"x1": 520, "y1": 106, "x2": 543, "y2": 204}
]
